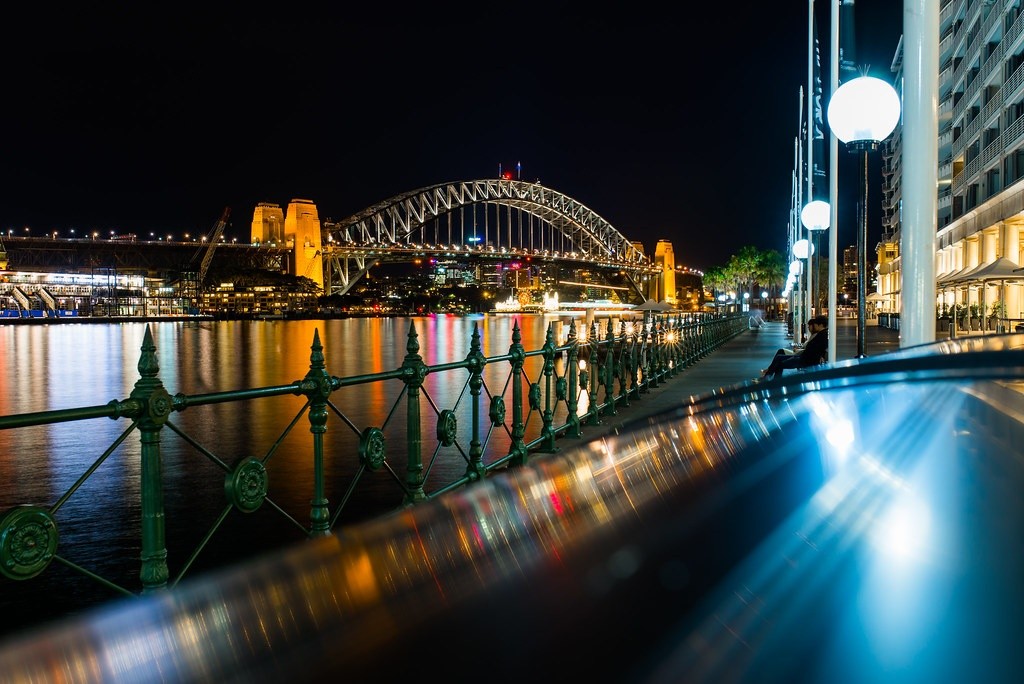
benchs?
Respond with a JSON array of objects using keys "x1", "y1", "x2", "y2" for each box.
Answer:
[{"x1": 797, "y1": 350, "x2": 829, "y2": 370}]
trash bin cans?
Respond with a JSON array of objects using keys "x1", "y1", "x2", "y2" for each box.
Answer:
[
  {"x1": 995, "y1": 325, "x2": 1005, "y2": 333},
  {"x1": 949, "y1": 323, "x2": 954, "y2": 339},
  {"x1": 787, "y1": 311, "x2": 793, "y2": 334}
]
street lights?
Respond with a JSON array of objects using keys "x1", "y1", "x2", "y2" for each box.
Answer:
[
  {"x1": 827, "y1": 75, "x2": 901, "y2": 357},
  {"x1": 761, "y1": 291, "x2": 768, "y2": 319},
  {"x1": 801, "y1": 200, "x2": 830, "y2": 314},
  {"x1": 782, "y1": 260, "x2": 804, "y2": 335},
  {"x1": 792, "y1": 238, "x2": 815, "y2": 344},
  {"x1": 744, "y1": 293, "x2": 749, "y2": 313}
]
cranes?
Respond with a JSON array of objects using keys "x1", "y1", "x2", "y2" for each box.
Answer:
[
  {"x1": 188, "y1": 207, "x2": 230, "y2": 285},
  {"x1": 291, "y1": 250, "x2": 321, "y2": 306}
]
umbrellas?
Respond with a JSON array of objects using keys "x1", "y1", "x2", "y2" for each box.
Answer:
[
  {"x1": 885, "y1": 289, "x2": 901, "y2": 313},
  {"x1": 659, "y1": 300, "x2": 676, "y2": 312},
  {"x1": 852, "y1": 292, "x2": 890, "y2": 313},
  {"x1": 935, "y1": 256, "x2": 1024, "y2": 335},
  {"x1": 633, "y1": 299, "x2": 664, "y2": 318}
]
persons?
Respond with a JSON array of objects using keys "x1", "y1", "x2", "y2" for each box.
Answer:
[{"x1": 750, "y1": 314, "x2": 828, "y2": 383}]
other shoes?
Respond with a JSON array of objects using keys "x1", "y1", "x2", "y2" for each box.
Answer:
[
  {"x1": 761, "y1": 370, "x2": 768, "y2": 374},
  {"x1": 751, "y1": 378, "x2": 760, "y2": 383}
]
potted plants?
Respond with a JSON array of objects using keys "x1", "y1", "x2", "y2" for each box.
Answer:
[{"x1": 936, "y1": 300, "x2": 1007, "y2": 332}]
